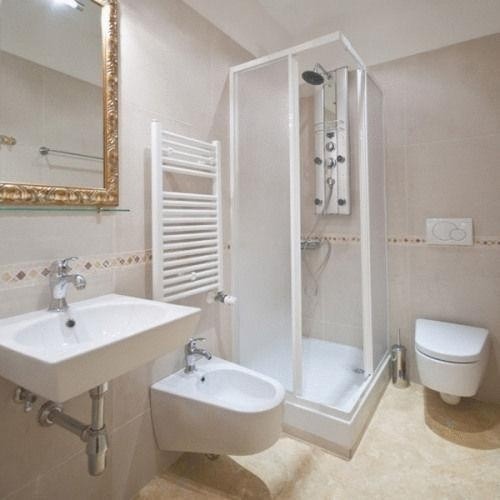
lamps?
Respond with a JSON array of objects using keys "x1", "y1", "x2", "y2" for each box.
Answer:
[{"x1": 62, "y1": 0, "x2": 85, "y2": 12}]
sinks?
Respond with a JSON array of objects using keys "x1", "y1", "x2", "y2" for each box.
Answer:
[
  {"x1": 0, "y1": 294, "x2": 203, "y2": 403},
  {"x1": 151, "y1": 356, "x2": 285, "y2": 455}
]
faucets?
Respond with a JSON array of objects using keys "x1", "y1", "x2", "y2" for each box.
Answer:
[
  {"x1": 184, "y1": 337, "x2": 211, "y2": 374},
  {"x1": 48, "y1": 257, "x2": 86, "y2": 311}
]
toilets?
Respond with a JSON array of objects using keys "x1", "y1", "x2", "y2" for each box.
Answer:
[{"x1": 414, "y1": 320, "x2": 490, "y2": 406}]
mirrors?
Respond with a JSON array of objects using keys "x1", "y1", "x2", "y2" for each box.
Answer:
[{"x1": 0, "y1": 0, "x2": 121, "y2": 209}]
